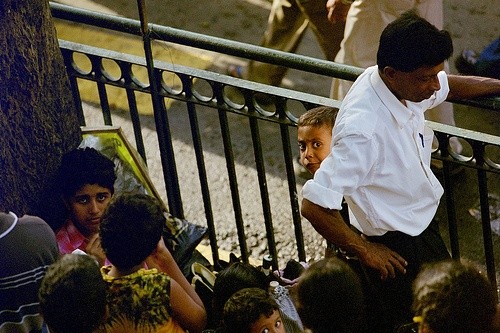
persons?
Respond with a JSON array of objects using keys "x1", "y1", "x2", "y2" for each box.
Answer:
[
  {"x1": 327, "y1": 0, "x2": 462, "y2": 168},
  {"x1": 54, "y1": 147, "x2": 117, "y2": 276},
  {"x1": 300, "y1": 9, "x2": 500, "y2": 319},
  {"x1": 456, "y1": 35, "x2": 500, "y2": 103},
  {"x1": 227, "y1": 0, "x2": 357, "y2": 103},
  {"x1": 297, "y1": 105, "x2": 362, "y2": 269},
  {"x1": 0, "y1": 193, "x2": 500, "y2": 333}
]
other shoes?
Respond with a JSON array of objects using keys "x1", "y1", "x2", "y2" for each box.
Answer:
[
  {"x1": 456, "y1": 49, "x2": 481, "y2": 77},
  {"x1": 227, "y1": 63, "x2": 274, "y2": 106}
]
